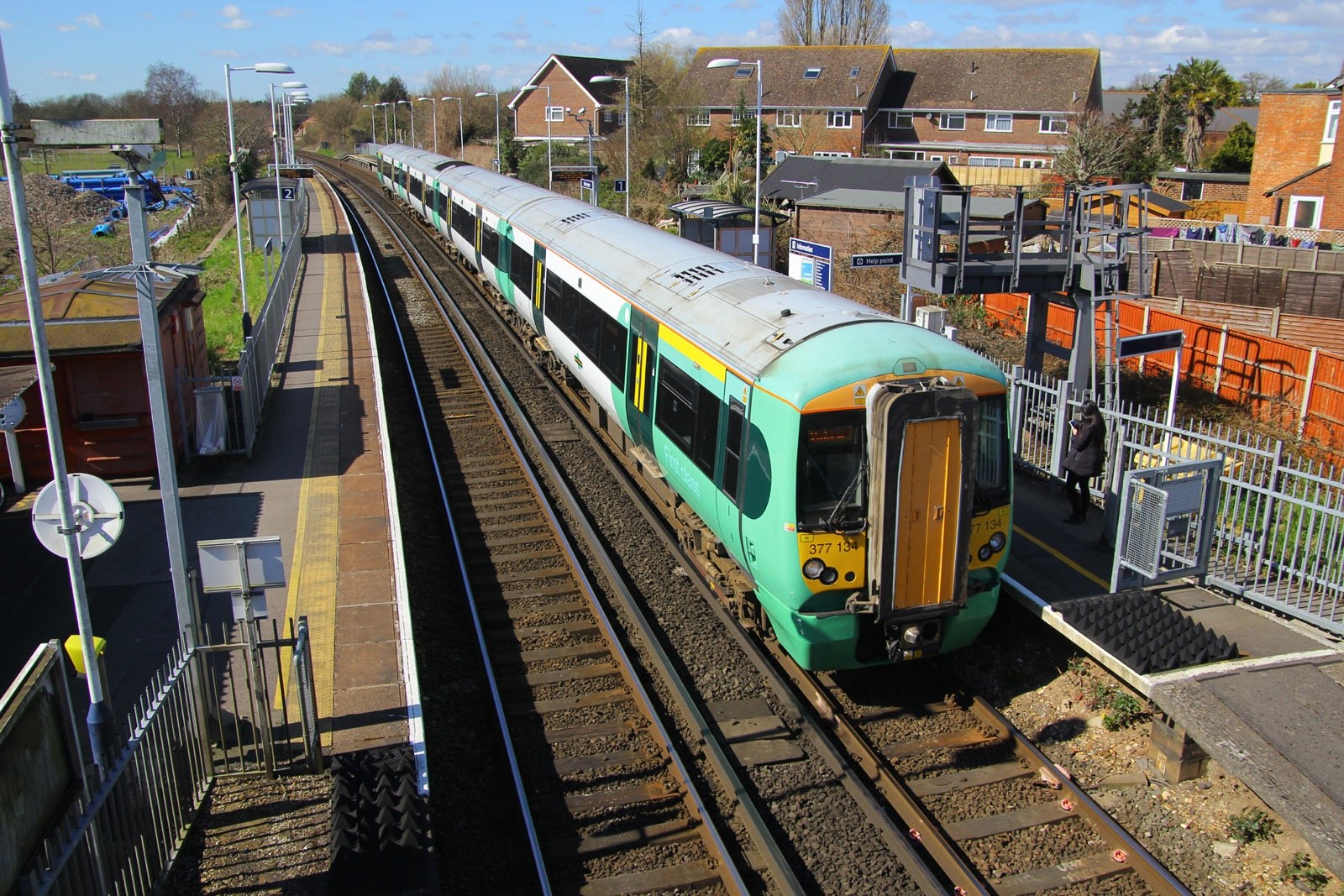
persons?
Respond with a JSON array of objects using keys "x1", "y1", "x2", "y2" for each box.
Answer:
[{"x1": 1062, "y1": 400, "x2": 1107, "y2": 523}]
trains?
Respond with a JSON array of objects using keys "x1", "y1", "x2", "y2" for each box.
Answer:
[{"x1": 374, "y1": 141, "x2": 1015, "y2": 676}]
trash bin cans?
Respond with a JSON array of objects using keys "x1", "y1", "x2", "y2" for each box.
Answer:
[{"x1": 193, "y1": 386, "x2": 226, "y2": 456}]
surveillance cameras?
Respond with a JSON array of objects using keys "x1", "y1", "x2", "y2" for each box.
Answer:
[
  {"x1": 230, "y1": 154, "x2": 237, "y2": 167},
  {"x1": 109, "y1": 144, "x2": 155, "y2": 164},
  {"x1": 278, "y1": 137, "x2": 285, "y2": 142},
  {"x1": 238, "y1": 148, "x2": 250, "y2": 156},
  {"x1": 272, "y1": 131, "x2": 278, "y2": 139}
]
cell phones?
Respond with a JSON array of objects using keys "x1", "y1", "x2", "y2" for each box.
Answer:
[{"x1": 1069, "y1": 420, "x2": 1075, "y2": 428}]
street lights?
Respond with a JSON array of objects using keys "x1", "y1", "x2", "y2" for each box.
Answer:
[
  {"x1": 362, "y1": 104, "x2": 376, "y2": 144},
  {"x1": 268, "y1": 80, "x2": 308, "y2": 255},
  {"x1": 397, "y1": 100, "x2": 415, "y2": 148},
  {"x1": 418, "y1": 97, "x2": 437, "y2": 154},
  {"x1": 520, "y1": 85, "x2": 552, "y2": 191},
  {"x1": 223, "y1": 61, "x2": 296, "y2": 345},
  {"x1": 374, "y1": 103, "x2": 389, "y2": 145},
  {"x1": 277, "y1": 91, "x2": 312, "y2": 194},
  {"x1": 475, "y1": 92, "x2": 501, "y2": 174},
  {"x1": 706, "y1": 57, "x2": 763, "y2": 265},
  {"x1": 381, "y1": 102, "x2": 397, "y2": 144},
  {"x1": 441, "y1": 97, "x2": 464, "y2": 162},
  {"x1": 589, "y1": 76, "x2": 630, "y2": 218}
]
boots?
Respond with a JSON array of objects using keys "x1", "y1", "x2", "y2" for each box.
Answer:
[
  {"x1": 1063, "y1": 496, "x2": 1081, "y2": 524},
  {"x1": 1081, "y1": 495, "x2": 1090, "y2": 522}
]
